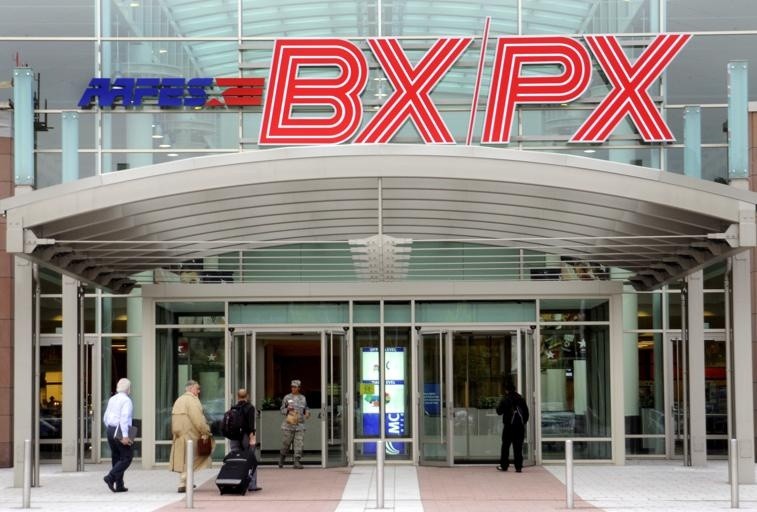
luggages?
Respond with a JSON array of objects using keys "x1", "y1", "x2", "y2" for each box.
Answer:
[{"x1": 215, "y1": 442, "x2": 260, "y2": 495}]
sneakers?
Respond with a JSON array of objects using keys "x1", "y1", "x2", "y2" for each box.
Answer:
[
  {"x1": 104, "y1": 476, "x2": 116, "y2": 491},
  {"x1": 248, "y1": 486, "x2": 261, "y2": 491},
  {"x1": 179, "y1": 485, "x2": 186, "y2": 492},
  {"x1": 516, "y1": 467, "x2": 521, "y2": 472},
  {"x1": 496, "y1": 465, "x2": 503, "y2": 470},
  {"x1": 116, "y1": 486, "x2": 128, "y2": 491}
]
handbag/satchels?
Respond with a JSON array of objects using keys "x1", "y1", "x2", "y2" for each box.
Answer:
[
  {"x1": 223, "y1": 450, "x2": 247, "y2": 463},
  {"x1": 197, "y1": 437, "x2": 211, "y2": 456}
]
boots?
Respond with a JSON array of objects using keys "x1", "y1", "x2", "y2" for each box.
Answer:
[
  {"x1": 279, "y1": 454, "x2": 285, "y2": 467},
  {"x1": 295, "y1": 457, "x2": 303, "y2": 469}
]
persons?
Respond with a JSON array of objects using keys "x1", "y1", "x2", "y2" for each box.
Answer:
[
  {"x1": 496, "y1": 382, "x2": 529, "y2": 473},
  {"x1": 230, "y1": 389, "x2": 263, "y2": 491},
  {"x1": 46, "y1": 396, "x2": 58, "y2": 415},
  {"x1": 168, "y1": 380, "x2": 213, "y2": 492},
  {"x1": 102, "y1": 377, "x2": 135, "y2": 492},
  {"x1": 278, "y1": 380, "x2": 311, "y2": 469}
]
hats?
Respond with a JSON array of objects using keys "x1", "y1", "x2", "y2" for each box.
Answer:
[{"x1": 291, "y1": 381, "x2": 301, "y2": 387}]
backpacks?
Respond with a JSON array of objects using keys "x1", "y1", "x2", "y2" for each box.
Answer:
[
  {"x1": 222, "y1": 403, "x2": 247, "y2": 440},
  {"x1": 510, "y1": 407, "x2": 524, "y2": 425}
]
